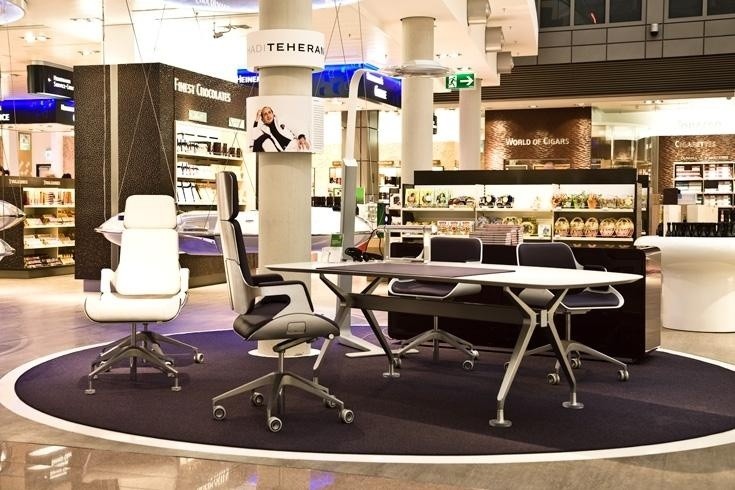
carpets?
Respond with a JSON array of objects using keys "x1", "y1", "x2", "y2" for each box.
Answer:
[{"x1": 0, "y1": 322, "x2": 735, "y2": 466}]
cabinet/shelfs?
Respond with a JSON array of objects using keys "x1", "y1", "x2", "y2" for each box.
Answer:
[
  {"x1": 0, "y1": 175, "x2": 76, "y2": 280},
  {"x1": 400, "y1": 166, "x2": 650, "y2": 251},
  {"x1": 173, "y1": 118, "x2": 246, "y2": 209},
  {"x1": 673, "y1": 161, "x2": 734, "y2": 206}
]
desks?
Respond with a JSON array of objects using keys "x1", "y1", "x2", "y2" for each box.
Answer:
[{"x1": 263, "y1": 256, "x2": 644, "y2": 429}]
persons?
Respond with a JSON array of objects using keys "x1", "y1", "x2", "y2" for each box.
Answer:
[{"x1": 250, "y1": 105, "x2": 312, "y2": 153}]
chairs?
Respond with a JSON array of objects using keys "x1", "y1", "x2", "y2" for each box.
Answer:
[
  {"x1": 83, "y1": 194, "x2": 205, "y2": 396},
  {"x1": 502, "y1": 241, "x2": 630, "y2": 386},
  {"x1": 387, "y1": 236, "x2": 483, "y2": 371},
  {"x1": 211, "y1": 170, "x2": 357, "y2": 433}
]
